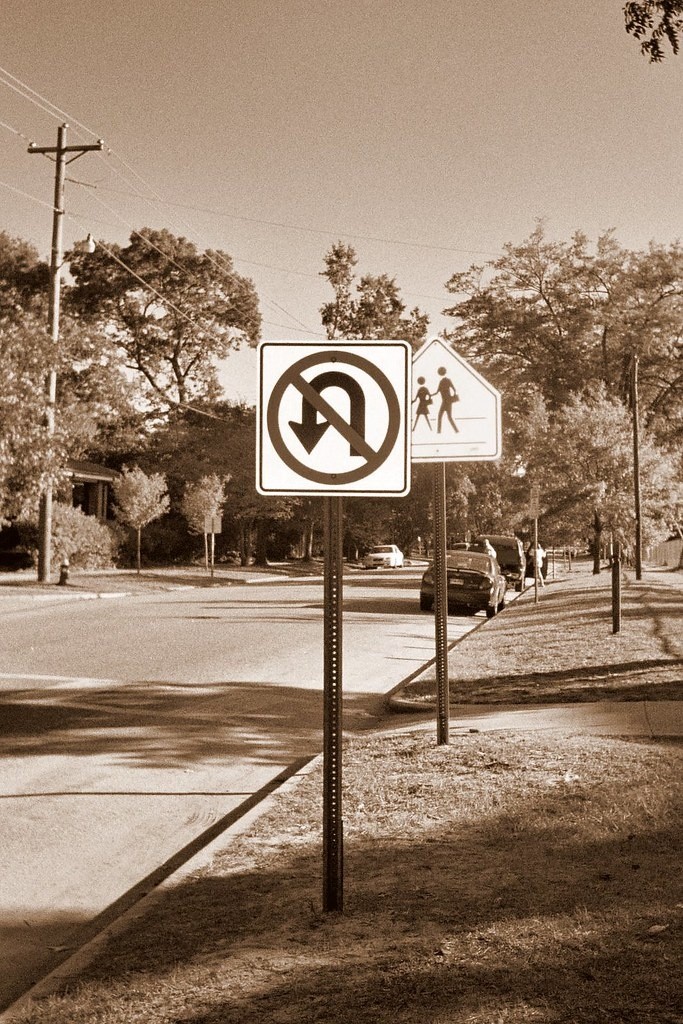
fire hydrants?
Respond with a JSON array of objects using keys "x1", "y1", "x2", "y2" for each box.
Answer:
[{"x1": 55, "y1": 556, "x2": 72, "y2": 587}]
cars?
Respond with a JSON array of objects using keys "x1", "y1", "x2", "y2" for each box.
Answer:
[
  {"x1": 418, "y1": 550, "x2": 511, "y2": 617},
  {"x1": 366, "y1": 544, "x2": 404, "y2": 569}
]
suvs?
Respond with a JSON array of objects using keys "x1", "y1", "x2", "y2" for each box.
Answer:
[{"x1": 453, "y1": 534, "x2": 549, "y2": 592}]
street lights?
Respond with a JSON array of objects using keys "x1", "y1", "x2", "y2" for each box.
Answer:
[{"x1": 35, "y1": 232, "x2": 97, "y2": 582}]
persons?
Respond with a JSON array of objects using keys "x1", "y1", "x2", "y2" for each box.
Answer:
[
  {"x1": 482, "y1": 538, "x2": 497, "y2": 559},
  {"x1": 529, "y1": 542, "x2": 547, "y2": 587},
  {"x1": 55, "y1": 551, "x2": 70, "y2": 586},
  {"x1": 587, "y1": 538, "x2": 599, "y2": 574}
]
